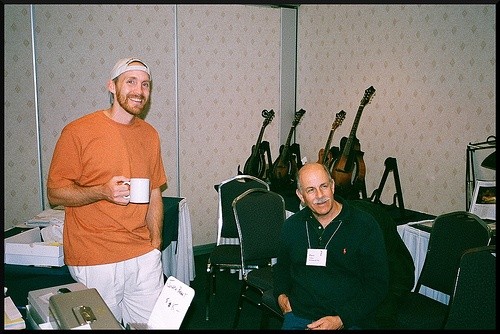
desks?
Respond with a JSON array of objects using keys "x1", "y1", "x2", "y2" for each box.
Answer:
[
  {"x1": 4, "y1": 197, "x2": 197, "y2": 330},
  {"x1": 396, "y1": 225, "x2": 451, "y2": 306}
]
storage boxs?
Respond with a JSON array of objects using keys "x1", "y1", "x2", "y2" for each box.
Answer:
[
  {"x1": 26, "y1": 282, "x2": 125, "y2": 330},
  {"x1": 5, "y1": 227, "x2": 64, "y2": 267}
]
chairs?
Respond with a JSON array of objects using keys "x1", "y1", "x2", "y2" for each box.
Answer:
[
  {"x1": 394, "y1": 211, "x2": 492, "y2": 330},
  {"x1": 443, "y1": 244, "x2": 498, "y2": 330},
  {"x1": 227, "y1": 187, "x2": 286, "y2": 329},
  {"x1": 206, "y1": 175, "x2": 271, "y2": 321}
]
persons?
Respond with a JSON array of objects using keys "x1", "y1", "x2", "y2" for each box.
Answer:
[
  {"x1": 272, "y1": 162, "x2": 388, "y2": 330},
  {"x1": 47, "y1": 58, "x2": 166, "y2": 329}
]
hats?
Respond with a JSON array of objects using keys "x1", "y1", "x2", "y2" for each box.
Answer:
[{"x1": 110, "y1": 57, "x2": 151, "y2": 104}]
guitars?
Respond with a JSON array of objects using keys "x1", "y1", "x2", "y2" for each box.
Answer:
[
  {"x1": 268, "y1": 107, "x2": 307, "y2": 186},
  {"x1": 316, "y1": 110, "x2": 347, "y2": 168},
  {"x1": 329, "y1": 85, "x2": 377, "y2": 192},
  {"x1": 238, "y1": 109, "x2": 275, "y2": 193}
]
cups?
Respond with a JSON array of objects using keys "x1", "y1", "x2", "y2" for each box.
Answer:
[{"x1": 122, "y1": 178, "x2": 150, "y2": 204}]
